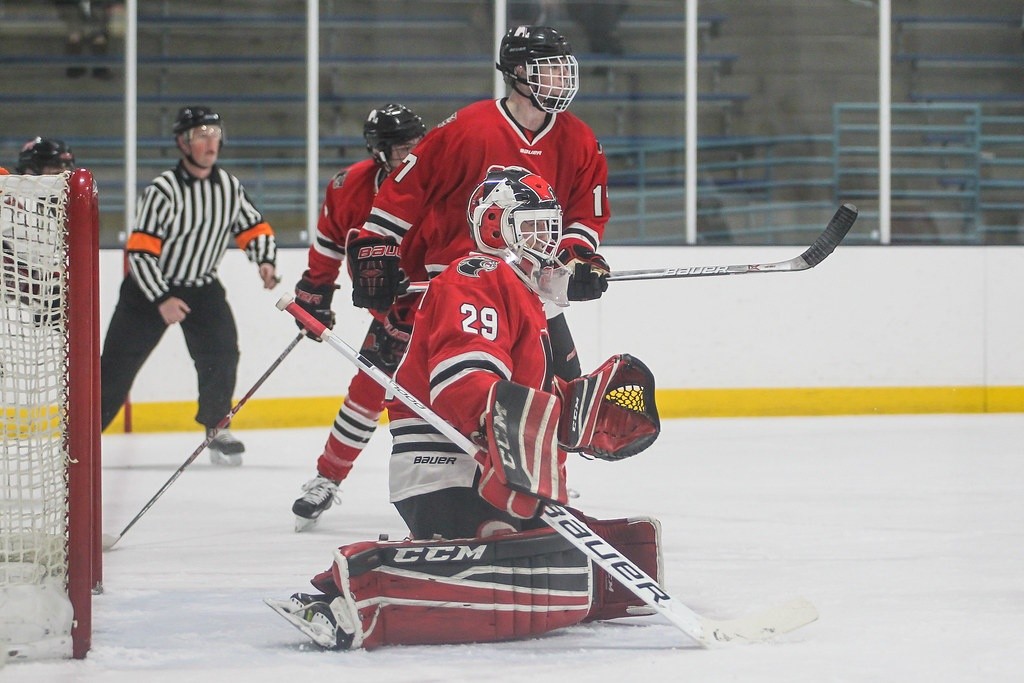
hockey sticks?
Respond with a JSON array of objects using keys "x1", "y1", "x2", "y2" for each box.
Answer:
[
  {"x1": 401, "y1": 200, "x2": 860, "y2": 294},
  {"x1": 102, "y1": 326, "x2": 304, "y2": 550},
  {"x1": 274, "y1": 292, "x2": 822, "y2": 651}
]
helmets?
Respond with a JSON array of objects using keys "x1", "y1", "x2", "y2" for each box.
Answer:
[
  {"x1": 17, "y1": 138, "x2": 76, "y2": 165},
  {"x1": 496, "y1": 24, "x2": 579, "y2": 113},
  {"x1": 467, "y1": 170, "x2": 572, "y2": 307},
  {"x1": 363, "y1": 105, "x2": 425, "y2": 174},
  {"x1": 172, "y1": 106, "x2": 220, "y2": 133}
]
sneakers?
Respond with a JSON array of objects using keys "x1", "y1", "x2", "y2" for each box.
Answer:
[
  {"x1": 292, "y1": 475, "x2": 339, "y2": 519},
  {"x1": 289, "y1": 592, "x2": 354, "y2": 651},
  {"x1": 207, "y1": 428, "x2": 244, "y2": 455}
]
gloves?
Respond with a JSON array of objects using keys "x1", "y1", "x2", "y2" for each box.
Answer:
[
  {"x1": 350, "y1": 241, "x2": 402, "y2": 314},
  {"x1": 295, "y1": 279, "x2": 341, "y2": 342},
  {"x1": 555, "y1": 245, "x2": 608, "y2": 301}
]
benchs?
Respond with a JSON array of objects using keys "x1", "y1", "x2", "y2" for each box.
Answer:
[
  {"x1": 0, "y1": 0, "x2": 770, "y2": 244},
  {"x1": 885, "y1": 0, "x2": 1024, "y2": 245}
]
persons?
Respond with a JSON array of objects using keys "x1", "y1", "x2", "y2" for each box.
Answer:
[
  {"x1": 101, "y1": 105, "x2": 278, "y2": 457},
  {"x1": 289, "y1": 24, "x2": 613, "y2": 648},
  {"x1": 0, "y1": 136, "x2": 76, "y2": 334}
]
